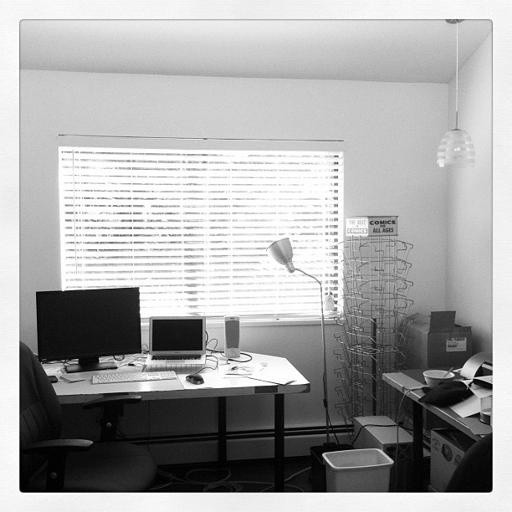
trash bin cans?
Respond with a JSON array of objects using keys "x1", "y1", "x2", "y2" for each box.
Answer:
[{"x1": 322, "y1": 447, "x2": 395, "y2": 493}]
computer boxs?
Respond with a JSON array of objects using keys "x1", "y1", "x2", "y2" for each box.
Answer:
[{"x1": 351, "y1": 415, "x2": 414, "y2": 493}]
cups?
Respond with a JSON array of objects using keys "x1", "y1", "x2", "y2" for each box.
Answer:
[{"x1": 479, "y1": 396, "x2": 490, "y2": 426}]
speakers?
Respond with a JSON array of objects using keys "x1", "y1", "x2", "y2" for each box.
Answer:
[{"x1": 223, "y1": 315, "x2": 241, "y2": 359}]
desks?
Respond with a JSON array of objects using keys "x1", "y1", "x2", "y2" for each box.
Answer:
[
  {"x1": 33, "y1": 351, "x2": 310, "y2": 492},
  {"x1": 381, "y1": 369, "x2": 492, "y2": 492}
]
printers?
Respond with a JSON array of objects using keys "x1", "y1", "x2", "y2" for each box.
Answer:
[{"x1": 460, "y1": 352, "x2": 493, "y2": 421}]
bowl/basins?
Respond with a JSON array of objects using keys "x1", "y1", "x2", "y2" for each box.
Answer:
[{"x1": 421, "y1": 370, "x2": 455, "y2": 388}]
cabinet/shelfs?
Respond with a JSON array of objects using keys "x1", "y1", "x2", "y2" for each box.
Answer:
[{"x1": 333, "y1": 236, "x2": 413, "y2": 444}]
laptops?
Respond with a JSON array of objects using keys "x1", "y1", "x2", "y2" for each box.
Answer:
[{"x1": 146, "y1": 315, "x2": 207, "y2": 368}]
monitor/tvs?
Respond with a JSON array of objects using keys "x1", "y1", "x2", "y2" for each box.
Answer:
[{"x1": 36, "y1": 287, "x2": 143, "y2": 372}]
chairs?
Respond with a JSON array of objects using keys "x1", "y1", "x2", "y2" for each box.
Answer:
[{"x1": 20, "y1": 341, "x2": 155, "y2": 492}]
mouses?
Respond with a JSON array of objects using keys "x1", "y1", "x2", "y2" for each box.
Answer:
[{"x1": 185, "y1": 373, "x2": 204, "y2": 384}]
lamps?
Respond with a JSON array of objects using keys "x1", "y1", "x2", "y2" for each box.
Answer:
[
  {"x1": 267, "y1": 238, "x2": 354, "y2": 477},
  {"x1": 436, "y1": 19, "x2": 476, "y2": 168}
]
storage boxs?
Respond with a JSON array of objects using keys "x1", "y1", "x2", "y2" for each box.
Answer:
[{"x1": 400, "y1": 311, "x2": 474, "y2": 370}]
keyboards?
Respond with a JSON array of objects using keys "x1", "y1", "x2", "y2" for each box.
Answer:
[{"x1": 92, "y1": 370, "x2": 179, "y2": 385}]
